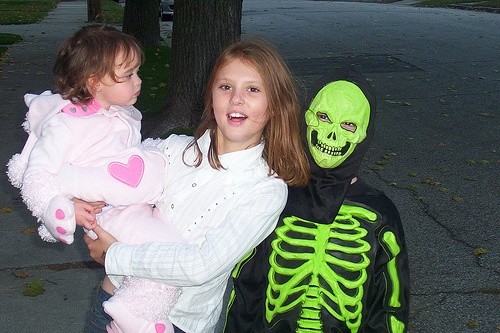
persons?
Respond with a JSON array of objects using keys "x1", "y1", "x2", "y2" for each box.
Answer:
[
  {"x1": 6, "y1": 23, "x2": 183, "y2": 333},
  {"x1": 223, "y1": 69, "x2": 411, "y2": 333},
  {"x1": 84, "y1": 37, "x2": 310, "y2": 333}
]
